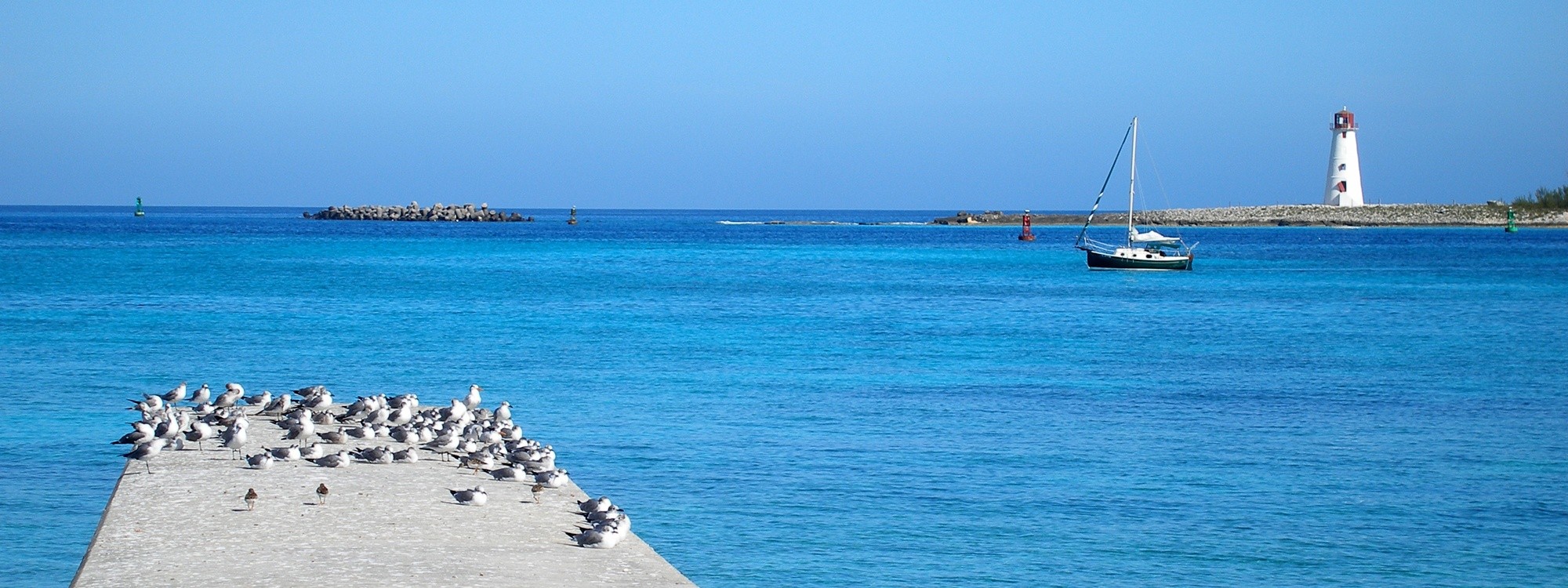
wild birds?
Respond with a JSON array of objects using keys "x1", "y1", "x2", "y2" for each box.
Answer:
[{"x1": 107, "y1": 380, "x2": 631, "y2": 549}]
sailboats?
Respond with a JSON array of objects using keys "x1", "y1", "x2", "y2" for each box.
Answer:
[{"x1": 1073, "y1": 116, "x2": 1202, "y2": 272}]
